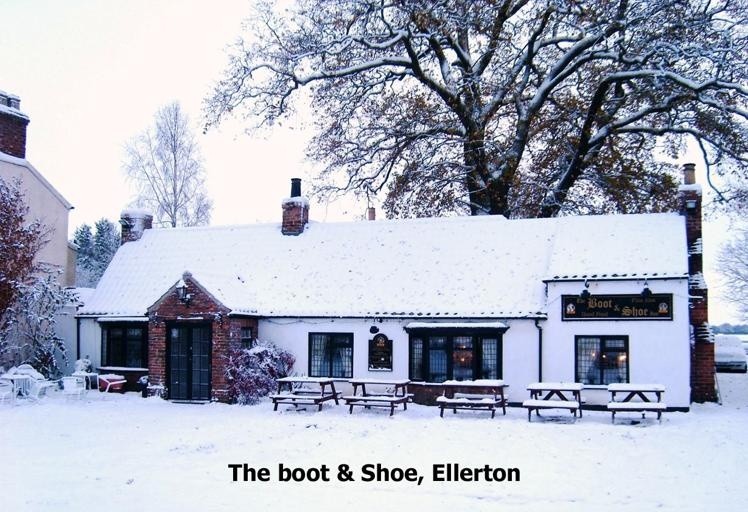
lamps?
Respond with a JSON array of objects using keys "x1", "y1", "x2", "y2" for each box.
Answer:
[
  {"x1": 370, "y1": 316, "x2": 383, "y2": 334},
  {"x1": 640, "y1": 277, "x2": 652, "y2": 300},
  {"x1": 175, "y1": 279, "x2": 192, "y2": 308},
  {"x1": 580, "y1": 278, "x2": 591, "y2": 300}
]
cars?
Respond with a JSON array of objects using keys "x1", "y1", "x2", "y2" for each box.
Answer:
[{"x1": 714, "y1": 334, "x2": 748, "y2": 373}]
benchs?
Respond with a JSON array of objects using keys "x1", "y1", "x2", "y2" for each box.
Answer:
[
  {"x1": 267, "y1": 389, "x2": 342, "y2": 414},
  {"x1": 607, "y1": 398, "x2": 668, "y2": 425},
  {"x1": 437, "y1": 393, "x2": 508, "y2": 419},
  {"x1": 342, "y1": 392, "x2": 414, "y2": 418},
  {"x1": 523, "y1": 397, "x2": 582, "y2": 423}
]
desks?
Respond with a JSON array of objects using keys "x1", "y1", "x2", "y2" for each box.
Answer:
[
  {"x1": 440, "y1": 380, "x2": 508, "y2": 419},
  {"x1": 608, "y1": 383, "x2": 665, "y2": 423},
  {"x1": 526, "y1": 383, "x2": 584, "y2": 422},
  {"x1": 73, "y1": 372, "x2": 100, "y2": 396},
  {"x1": 277, "y1": 376, "x2": 339, "y2": 411},
  {"x1": 349, "y1": 379, "x2": 411, "y2": 416}
]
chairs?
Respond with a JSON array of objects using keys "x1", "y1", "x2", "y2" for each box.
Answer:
[{"x1": 0, "y1": 362, "x2": 86, "y2": 407}]
